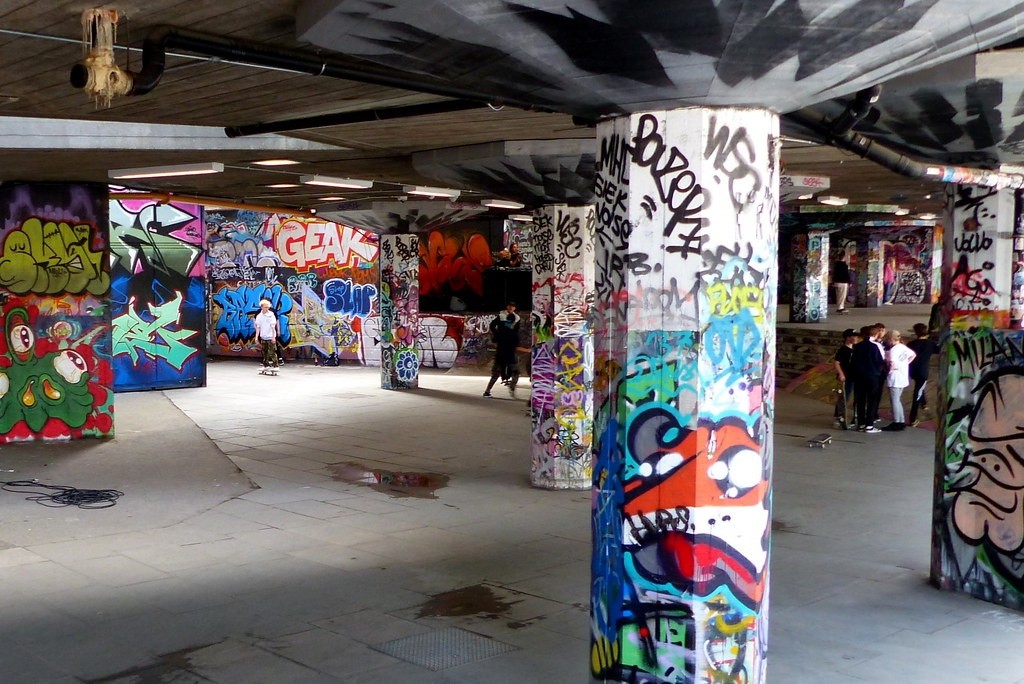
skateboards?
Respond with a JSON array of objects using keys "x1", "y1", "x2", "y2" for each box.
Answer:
[
  {"x1": 257, "y1": 367, "x2": 280, "y2": 376},
  {"x1": 831, "y1": 377, "x2": 847, "y2": 430},
  {"x1": 839, "y1": 310, "x2": 849, "y2": 315},
  {"x1": 807, "y1": 433, "x2": 832, "y2": 449}
]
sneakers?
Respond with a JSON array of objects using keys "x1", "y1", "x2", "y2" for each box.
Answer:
[
  {"x1": 922, "y1": 405, "x2": 929, "y2": 410},
  {"x1": 483, "y1": 392, "x2": 493, "y2": 398},
  {"x1": 865, "y1": 425, "x2": 883, "y2": 433},
  {"x1": 880, "y1": 422, "x2": 906, "y2": 431},
  {"x1": 910, "y1": 419, "x2": 920, "y2": 428},
  {"x1": 508, "y1": 387, "x2": 515, "y2": 398},
  {"x1": 858, "y1": 424, "x2": 866, "y2": 432}
]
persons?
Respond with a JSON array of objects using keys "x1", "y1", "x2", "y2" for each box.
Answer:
[
  {"x1": 832, "y1": 249, "x2": 851, "y2": 312},
  {"x1": 254, "y1": 299, "x2": 284, "y2": 370},
  {"x1": 882, "y1": 329, "x2": 916, "y2": 432},
  {"x1": 834, "y1": 322, "x2": 889, "y2": 434},
  {"x1": 906, "y1": 323, "x2": 941, "y2": 427},
  {"x1": 884, "y1": 255, "x2": 896, "y2": 305},
  {"x1": 484, "y1": 301, "x2": 522, "y2": 399}
]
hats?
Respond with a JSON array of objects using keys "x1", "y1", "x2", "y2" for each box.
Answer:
[{"x1": 843, "y1": 329, "x2": 860, "y2": 337}]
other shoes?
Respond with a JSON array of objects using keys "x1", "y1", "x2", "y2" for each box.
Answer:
[
  {"x1": 833, "y1": 417, "x2": 843, "y2": 427},
  {"x1": 258, "y1": 366, "x2": 281, "y2": 372},
  {"x1": 278, "y1": 358, "x2": 285, "y2": 366}
]
